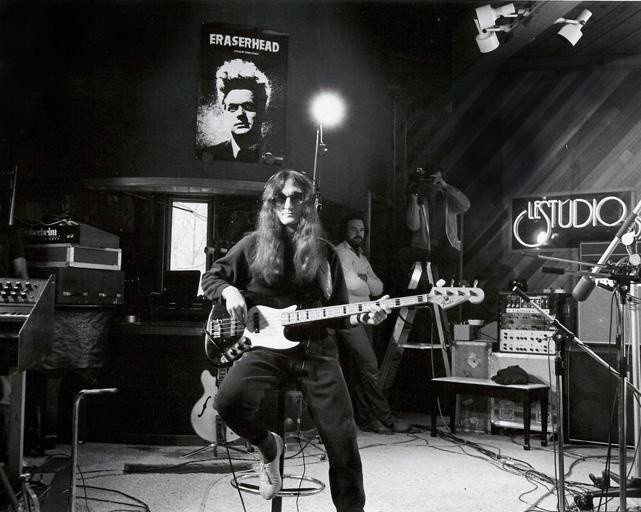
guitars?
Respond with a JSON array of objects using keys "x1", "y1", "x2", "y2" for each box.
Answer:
[
  {"x1": 204, "y1": 279, "x2": 485, "y2": 369},
  {"x1": 191, "y1": 368, "x2": 243, "y2": 444}
]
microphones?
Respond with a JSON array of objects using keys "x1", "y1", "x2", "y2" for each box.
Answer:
[
  {"x1": 203, "y1": 245, "x2": 228, "y2": 255},
  {"x1": 79, "y1": 387, "x2": 119, "y2": 396},
  {"x1": 571, "y1": 274, "x2": 596, "y2": 303}
]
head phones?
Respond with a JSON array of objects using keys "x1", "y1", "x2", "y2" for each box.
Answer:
[{"x1": 296, "y1": 169, "x2": 324, "y2": 212}]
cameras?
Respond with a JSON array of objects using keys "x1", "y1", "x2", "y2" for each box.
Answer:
[{"x1": 409, "y1": 165, "x2": 441, "y2": 197}]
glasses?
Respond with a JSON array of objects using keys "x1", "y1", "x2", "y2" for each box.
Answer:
[{"x1": 270, "y1": 192, "x2": 304, "y2": 209}]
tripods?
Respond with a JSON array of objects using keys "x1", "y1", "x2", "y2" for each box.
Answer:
[{"x1": 177, "y1": 340, "x2": 250, "y2": 461}]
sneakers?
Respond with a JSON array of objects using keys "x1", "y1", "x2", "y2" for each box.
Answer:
[
  {"x1": 256, "y1": 429, "x2": 285, "y2": 503},
  {"x1": 386, "y1": 420, "x2": 414, "y2": 434},
  {"x1": 359, "y1": 418, "x2": 394, "y2": 436}
]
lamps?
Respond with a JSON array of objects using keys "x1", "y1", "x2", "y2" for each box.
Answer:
[{"x1": 462, "y1": 3, "x2": 594, "y2": 57}]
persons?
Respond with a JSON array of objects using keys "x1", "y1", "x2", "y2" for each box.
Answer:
[
  {"x1": 335, "y1": 215, "x2": 411, "y2": 436},
  {"x1": 0, "y1": 223, "x2": 30, "y2": 405},
  {"x1": 203, "y1": 58, "x2": 284, "y2": 165},
  {"x1": 200, "y1": 170, "x2": 366, "y2": 511},
  {"x1": 406, "y1": 169, "x2": 471, "y2": 328}
]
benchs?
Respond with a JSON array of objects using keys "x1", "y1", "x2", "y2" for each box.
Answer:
[{"x1": 428, "y1": 371, "x2": 550, "y2": 449}]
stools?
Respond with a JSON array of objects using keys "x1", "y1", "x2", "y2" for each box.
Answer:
[{"x1": 222, "y1": 379, "x2": 325, "y2": 512}]
